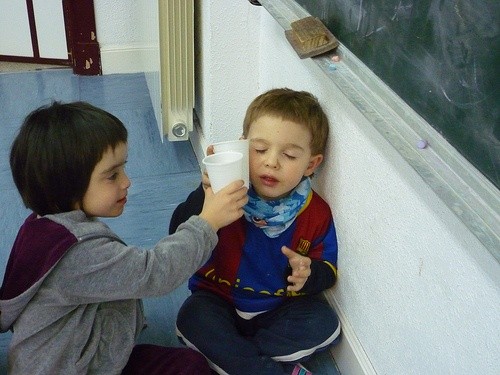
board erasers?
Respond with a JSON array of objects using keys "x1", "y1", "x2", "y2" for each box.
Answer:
[{"x1": 284, "y1": 16, "x2": 341, "y2": 61}]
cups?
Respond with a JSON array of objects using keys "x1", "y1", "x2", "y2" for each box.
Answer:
[
  {"x1": 202, "y1": 151, "x2": 243, "y2": 194},
  {"x1": 211, "y1": 139, "x2": 249, "y2": 189}
]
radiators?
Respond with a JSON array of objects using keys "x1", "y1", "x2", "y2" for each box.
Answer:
[{"x1": 139, "y1": 1, "x2": 196, "y2": 144}]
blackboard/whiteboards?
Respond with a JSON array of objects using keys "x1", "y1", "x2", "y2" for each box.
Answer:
[{"x1": 250, "y1": 0, "x2": 500, "y2": 296}]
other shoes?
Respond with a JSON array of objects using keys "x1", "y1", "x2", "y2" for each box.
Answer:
[{"x1": 291, "y1": 363, "x2": 312, "y2": 375}]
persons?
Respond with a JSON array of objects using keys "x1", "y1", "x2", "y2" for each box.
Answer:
[
  {"x1": 168, "y1": 88, "x2": 343, "y2": 375},
  {"x1": 0, "y1": 98, "x2": 249, "y2": 375}
]
